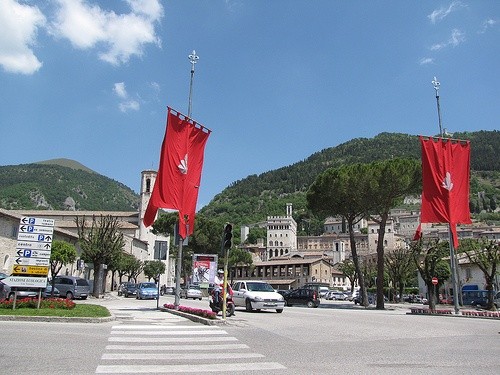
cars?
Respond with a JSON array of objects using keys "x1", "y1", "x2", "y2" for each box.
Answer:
[
  {"x1": 118, "y1": 283, "x2": 138, "y2": 297},
  {"x1": 397, "y1": 294, "x2": 427, "y2": 304},
  {"x1": 284, "y1": 289, "x2": 320, "y2": 307},
  {"x1": 231, "y1": 281, "x2": 284, "y2": 313},
  {"x1": 318, "y1": 287, "x2": 359, "y2": 302},
  {"x1": 0, "y1": 272, "x2": 39, "y2": 302},
  {"x1": 137, "y1": 282, "x2": 160, "y2": 299},
  {"x1": 160, "y1": 284, "x2": 175, "y2": 295},
  {"x1": 42, "y1": 283, "x2": 60, "y2": 299},
  {"x1": 276, "y1": 290, "x2": 291, "y2": 296},
  {"x1": 180, "y1": 285, "x2": 203, "y2": 301},
  {"x1": 49, "y1": 276, "x2": 89, "y2": 300}
]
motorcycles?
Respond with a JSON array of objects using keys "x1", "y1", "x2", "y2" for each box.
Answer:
[{"x1": 208, "y1": 281, "x2": 236, "y2": 317}]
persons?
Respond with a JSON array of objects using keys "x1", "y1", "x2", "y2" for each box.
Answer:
[{"x1": 214, "y1": 269, "x2": 236, "y2": 316}]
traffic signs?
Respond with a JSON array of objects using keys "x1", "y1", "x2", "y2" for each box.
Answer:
[{"x1": 12, "y1": 216, "x2": 55, "y2": 277}]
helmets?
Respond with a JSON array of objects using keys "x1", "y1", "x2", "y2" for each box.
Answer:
[{"x1": 218, "y1": 269, "x2": 224, "y2": 275}]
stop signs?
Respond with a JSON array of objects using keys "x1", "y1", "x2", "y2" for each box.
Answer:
[{"x1": 432, "y1": 278, "x2": 438, "y2": 284}]
